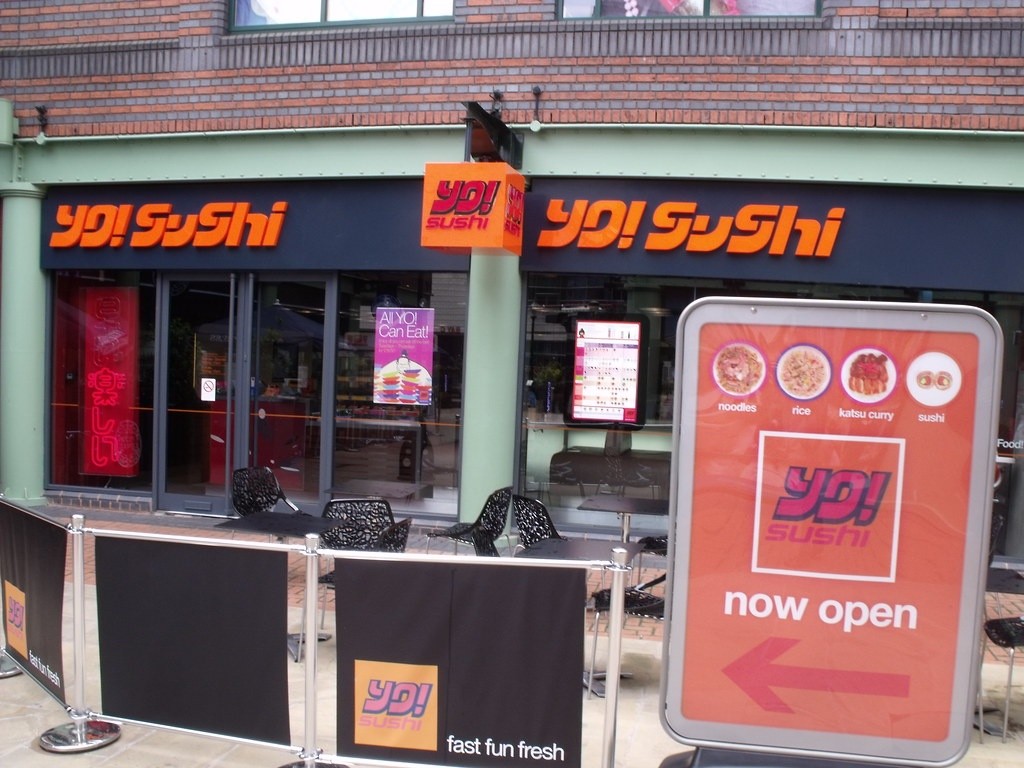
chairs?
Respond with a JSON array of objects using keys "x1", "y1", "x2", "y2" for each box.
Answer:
[
  {"x1": 229, "y1": 466, "x2": 299, "y2": 545},
  {"x1": 637, "y1": 535, "x2": 668, "y2": 585},
  {"x1": 975, "y1": 616, "x2": 1024, "y2": 744},
  {"x1": 425, "y1": 486, "x2": 513, "y2": 556},
  {"x1": 603, "y1": 448, "x2": 656, "y2": 499},
  {"x1": 509, "y1": 493, "x2": 568, "y2": 557},
  {"x1": 582, "y1": 573, "x2": 666, "y2": 701},
  {"x1": 373, "y1": 516, "x2": 413, "y2": 554},
  {"x1": 320, "y1": 498, "x2": 395, "y2": 551},
  {"x1": 538, "y1": 442, "x2": 585, "y2": 505}
]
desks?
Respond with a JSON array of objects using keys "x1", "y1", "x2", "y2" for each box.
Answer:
[
  {"x1": 514, "y1": 538, "x2": 646, "y2": 632},
  {"x1": 575, "y1": 495, "x2": 669, "y2": 566},
  {"x1": 322, "y1": 479, "x2": 428, "y2": 506},
  {"x1": 213, "y1": 510, "x2": 348, "y2": 545}
]
